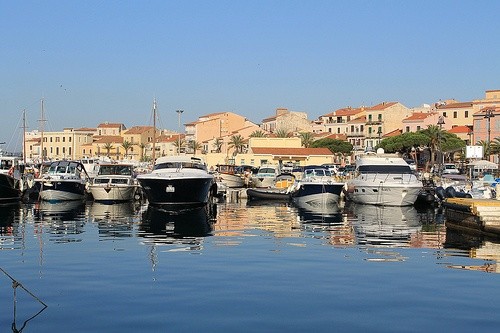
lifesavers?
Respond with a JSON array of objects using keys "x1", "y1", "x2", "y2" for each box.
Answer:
[{"x1": 8, "y1": 167, "x2": 13, "y2": 175}]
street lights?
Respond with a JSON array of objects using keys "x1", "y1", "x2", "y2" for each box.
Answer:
[
  {"x1": 483, "y1": 108, "x2": 496, "y2": 162},
  {"x1": 437, "y1": 115, "x2": 446, "y2": 153}
]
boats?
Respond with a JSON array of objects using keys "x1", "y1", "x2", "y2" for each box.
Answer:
[{"x1": 0, "y1": 96, "x2": 500, "y2": 215}]
